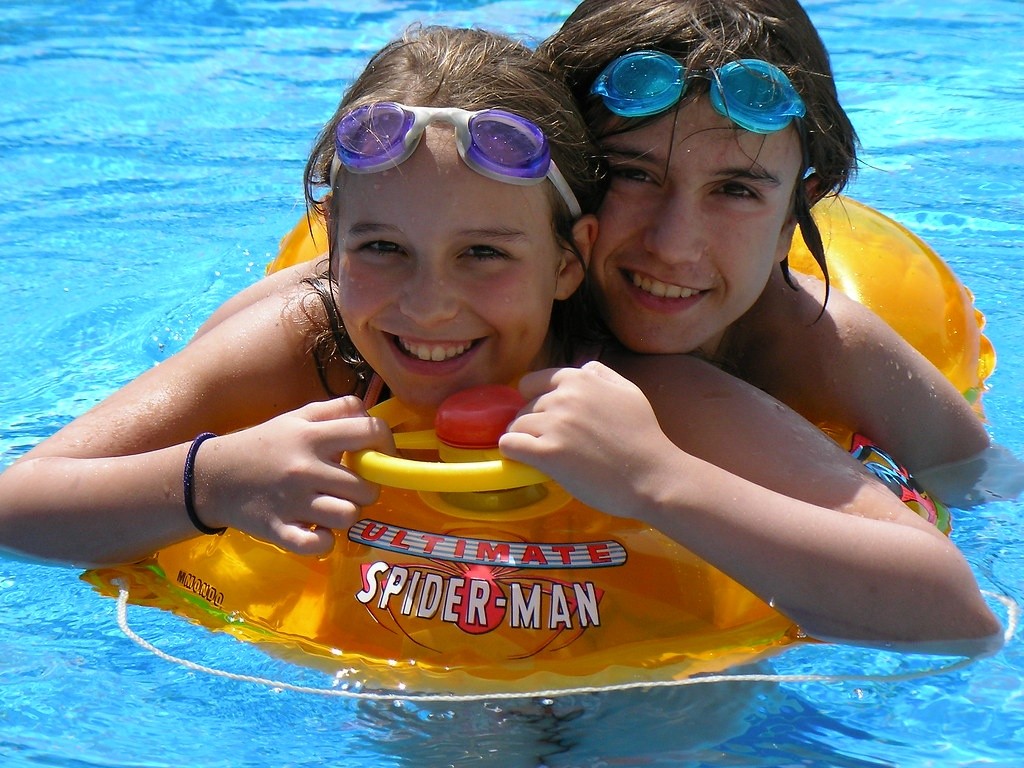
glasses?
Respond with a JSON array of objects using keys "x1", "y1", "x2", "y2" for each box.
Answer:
[
  {"x1": 581, "y1": 50, "x2": 813, "y2": 137},
  {"x1": 328, "y1": 100, "x2": 584, "y2": 220}
]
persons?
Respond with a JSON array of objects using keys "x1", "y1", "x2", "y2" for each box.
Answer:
[
  {"x1": 2, "y1": 23, "x2": 1006, "y2": 658},
  {"x1": 528, "y1": 1, "x2": 1023, "y2": 505}
]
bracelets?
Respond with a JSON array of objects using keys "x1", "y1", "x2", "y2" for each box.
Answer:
[{"x1": 179, "y1": 427, "x2": 225, "y2": 536}]
